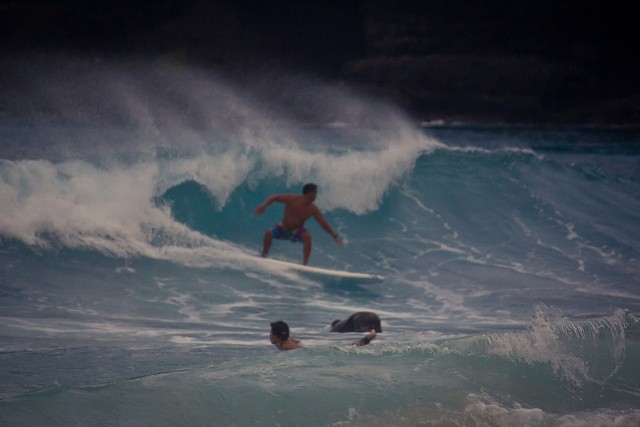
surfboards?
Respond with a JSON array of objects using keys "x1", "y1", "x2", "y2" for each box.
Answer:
[{"x1": 250, "y1": 257, "x2": 385, "y2": 283}]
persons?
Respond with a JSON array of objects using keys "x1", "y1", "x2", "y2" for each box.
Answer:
[
  {"x1": 254, "y1": 182, "x2": 343, "y2": 265},
  {"x1": 268, "y1": 319, "x2": 377, "y2": 350}
]
